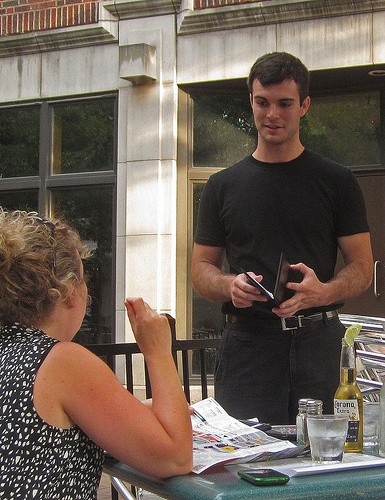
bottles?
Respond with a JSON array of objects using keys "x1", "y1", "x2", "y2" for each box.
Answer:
[
  {"x1": 379, "y1": 382, "x2": 385, "y2": 459},
  {"x1": 295, "y1": 398, "x2": 313, "y2": 445},
  {"x1": 333, "y1": 338, "x2": 364, "y2": 453},
  {"x1": 305, "y1": 400, "x2": 323, "y2": 444}
]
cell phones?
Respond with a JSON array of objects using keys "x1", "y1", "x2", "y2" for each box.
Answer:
[{"x1": 237, "y1": 468, "x2": 290, "y2": 486}]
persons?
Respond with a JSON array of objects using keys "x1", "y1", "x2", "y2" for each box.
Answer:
[
  {"x1": 193, "y1": 51, "x2": 373, "y2": 425},
  {"x1": 0, "y1": 208, "x2": 193, "y2": 500}
]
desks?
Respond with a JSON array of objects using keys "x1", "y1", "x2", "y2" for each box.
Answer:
[{"x1": 104, "y1": 440, "x2": 385, "y2": 499}]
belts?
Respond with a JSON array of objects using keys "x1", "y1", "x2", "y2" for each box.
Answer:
[{"x1": 226, "y1": 311, "x2": 338, "y2": 330}]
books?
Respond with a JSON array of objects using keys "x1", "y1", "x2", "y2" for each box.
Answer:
[{"x1": 240, "y1": 253, "x2": 301, "y2": 308}]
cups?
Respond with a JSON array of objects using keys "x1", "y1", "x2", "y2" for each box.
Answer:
[{"x1": 307, "y1": 415, "x2": 348, "y2": 465}]
